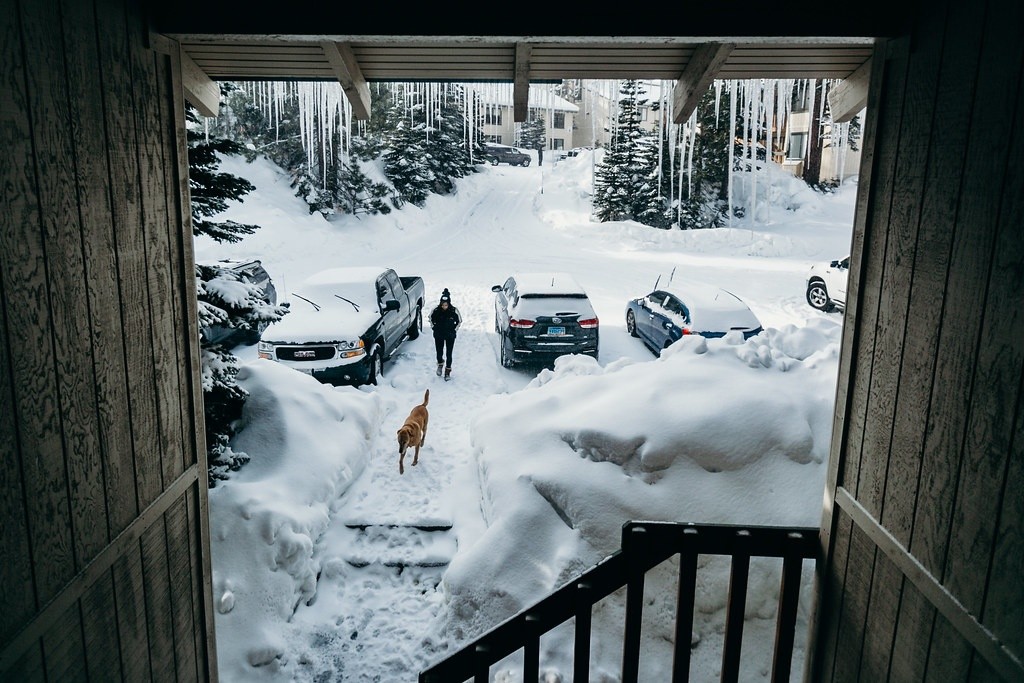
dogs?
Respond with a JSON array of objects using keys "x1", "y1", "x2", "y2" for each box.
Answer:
[{"x1": 397, "y1": 389, "x2": 430, "y2": 475}]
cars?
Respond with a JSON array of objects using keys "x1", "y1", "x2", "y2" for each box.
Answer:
[
  {"x1": 804, "y1": 254, "x2": 851, "y2": 314},
  {"x1": 196, "y1": 257, "x2": 281, "y2": 352},
  {"x1": 484, "y1": 146, "x2": 531, "y2": 167},
  {"x1": 624, "y1": 281, "x2": 764, "y2": 360}
]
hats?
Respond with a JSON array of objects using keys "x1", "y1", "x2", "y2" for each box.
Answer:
[{"x1": 440, "y1": 288, "x2": 451, "y2": 303}]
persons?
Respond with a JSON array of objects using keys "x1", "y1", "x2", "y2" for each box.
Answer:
[{"x1": 428, "y1": 287, "x2": 462, "y2": 382}]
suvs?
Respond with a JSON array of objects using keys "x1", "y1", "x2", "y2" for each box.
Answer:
[{"x1": 490, "y1": 271, "x2": 600, "y2": 366}]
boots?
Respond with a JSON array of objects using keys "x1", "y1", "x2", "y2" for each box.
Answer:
[
  {"x1": 436, "y1": 360, "x2": 444, "y2": 376},
  {"x1": 444, "y1": 366, "x2": 452, "y2": 381}
]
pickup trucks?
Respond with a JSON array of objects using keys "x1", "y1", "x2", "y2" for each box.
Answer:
[{"x1": 258, "y1": 266, "x2": 426, "y2": 388}]
